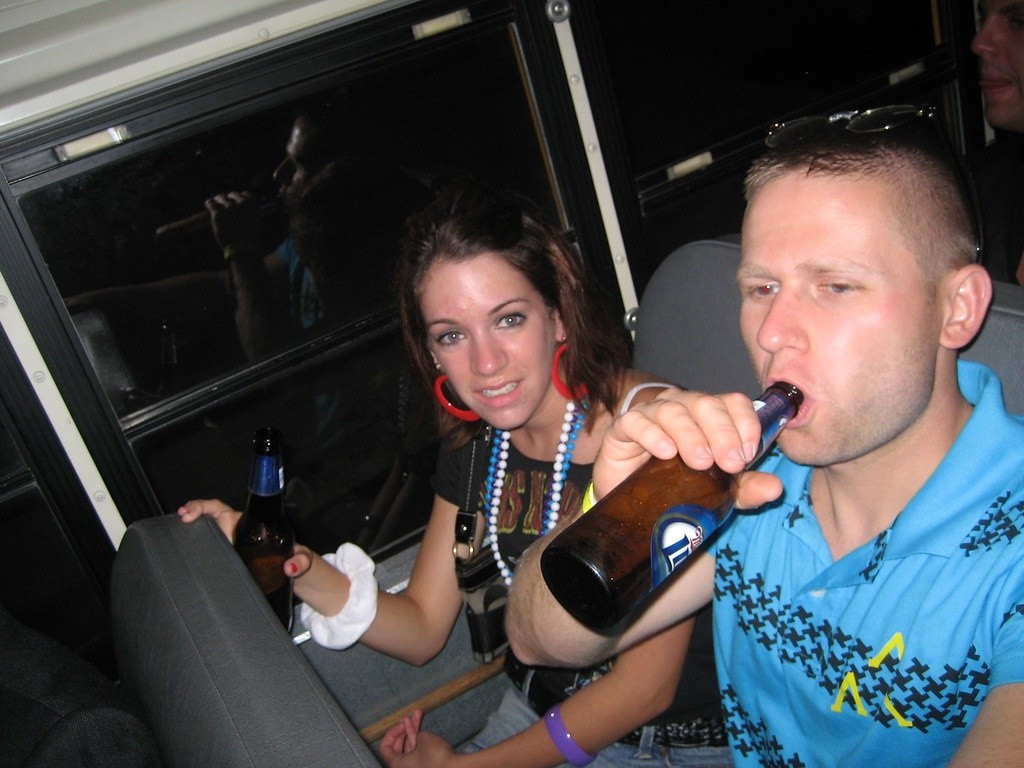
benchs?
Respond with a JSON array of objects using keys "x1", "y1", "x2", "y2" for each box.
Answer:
[
  {"x1": 635, "y1": 233, "x2": 1024, "y2": 422},
  {"x1": 108, "y1": 506, "x2": 389, "y2": 768}
]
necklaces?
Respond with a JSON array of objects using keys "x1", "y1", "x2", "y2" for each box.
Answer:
[
  {"x1": 397, "y1": 363, "x2": 410, "y2": 432},
  {"x1": 484, "y1": 395, "x2": 589, "y2": 588}
]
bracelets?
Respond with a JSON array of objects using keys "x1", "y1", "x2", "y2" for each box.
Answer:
[
  {"x1": 545, "y1": 703, "x2": 596, "y2": 768},
  {"x1": 582, "y1": 482, "x2": 597, "y2": 513},
  {"x1": 360, "y1": 515, "x2": 381, "y2": 529},
  {"x1": 300, "y1": 543, "x2": 378, "y2": 650},
  {"x1": 223, "y1": 239, "x2": 247, "y2": 261}
]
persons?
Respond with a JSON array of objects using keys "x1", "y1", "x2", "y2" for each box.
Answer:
[
  {"x1": 505, "y1": 102, "x2": 1024, "y2": 768},
  {"x1": 205, "y1": 95, "x2": 442, "y2": 560},
  {"x1": 178, "y1": 181, "x2": 735, "y2": 768},
  {"x1": 968, "y1": 0, "x2": 1024, "y2": 287}
]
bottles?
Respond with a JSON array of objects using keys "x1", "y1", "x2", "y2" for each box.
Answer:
[
  {"x1": 235, "y1": 436, "x2": 298, "y2": 635},
  {"x1": 540, "y1": 382, "x2": 804, "y2": 637}
]
